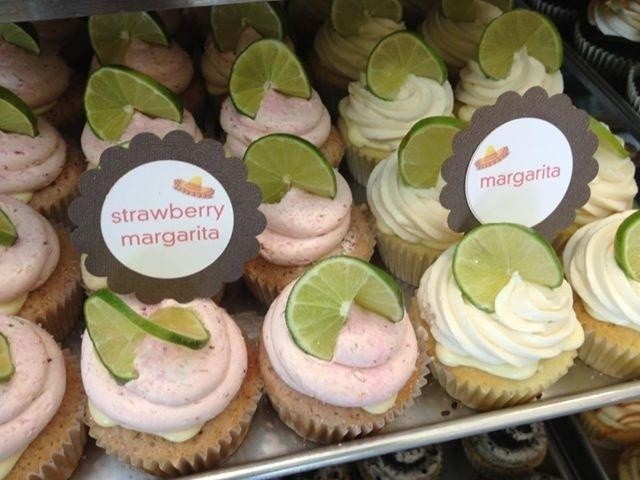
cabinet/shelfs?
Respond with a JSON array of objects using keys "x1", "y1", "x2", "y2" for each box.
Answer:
[{"x1": 0, "y1": 0, "x2": 640, "y2": 479}]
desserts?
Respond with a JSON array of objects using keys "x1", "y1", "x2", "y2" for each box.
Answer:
[{"x1": 0, "y1": 0, "x2": 639, "y2": 480}]
